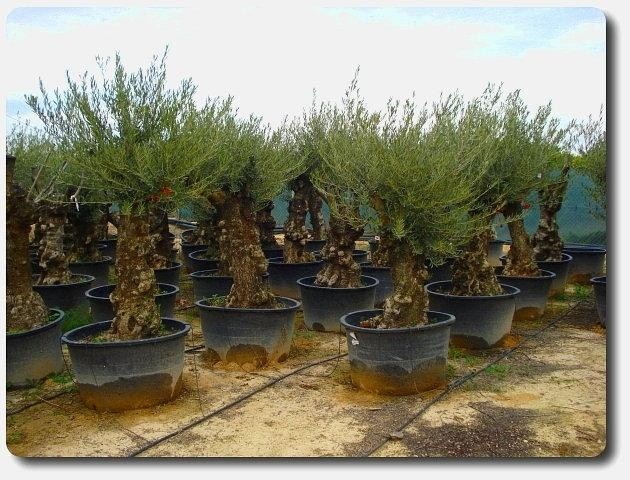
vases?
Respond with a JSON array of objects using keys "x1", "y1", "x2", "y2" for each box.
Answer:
[{"x1": 563, "y1": 243, "x2": 607, "y2": 329}]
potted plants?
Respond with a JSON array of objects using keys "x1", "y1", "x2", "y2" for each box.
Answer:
[
  {"x1": 5, "y1": 60, "x2": 572, "y2": 330},
  {"x1": 309, "y1": 116, "x2": 527, "y2": 398},
  {"x1": 419, "y1": 81, "x2": 524, "y2": 353},
  {"x1": 6, "y1": 106, "x2": 63, "y2": 389},
  {"x1": 15, "y1": 44, "x2": 262, "y2": 408},
  {"x1": 191, "y1": 91, "x2": 318, "y2": 368}
]
